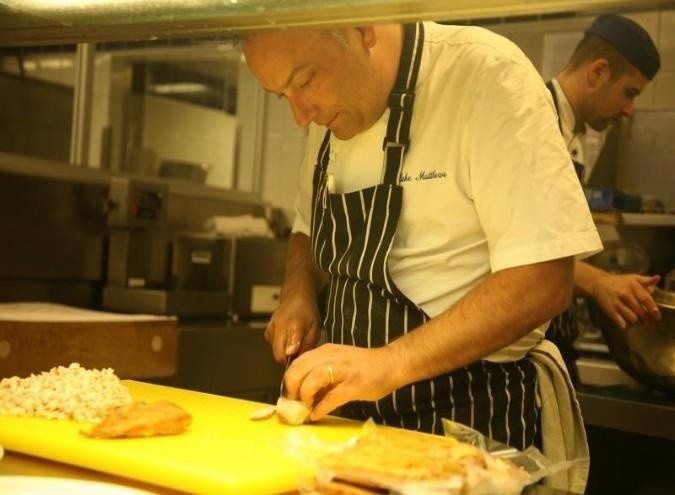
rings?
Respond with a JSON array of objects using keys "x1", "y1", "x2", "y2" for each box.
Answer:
[{"x1": 326, "y1": 364, "x2": 336, "y2": 386}]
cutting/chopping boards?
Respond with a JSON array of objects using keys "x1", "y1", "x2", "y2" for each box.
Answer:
[{"x1": 0, "y1": 376, "x2": 383, "y2": 495}]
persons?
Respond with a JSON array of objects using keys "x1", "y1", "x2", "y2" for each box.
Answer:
[
  {"x1": 238, "y1": 18, "x2": 605, "y2": 457},
  {"x1": 538, "y1": 13, "x2": 665, "y2": 420}
]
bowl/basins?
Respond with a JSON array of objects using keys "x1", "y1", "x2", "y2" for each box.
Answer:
[{"x1": 598, "y1": 287, "x2": 675, "y2": 392}]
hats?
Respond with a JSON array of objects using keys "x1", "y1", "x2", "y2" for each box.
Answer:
[{"x1": 584, "y1": 11, "x2": 662, "y2": 82}]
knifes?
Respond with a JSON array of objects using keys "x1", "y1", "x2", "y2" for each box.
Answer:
[{"x1": 279, "y1": 354, "x2": 295, "y2": 397}]
